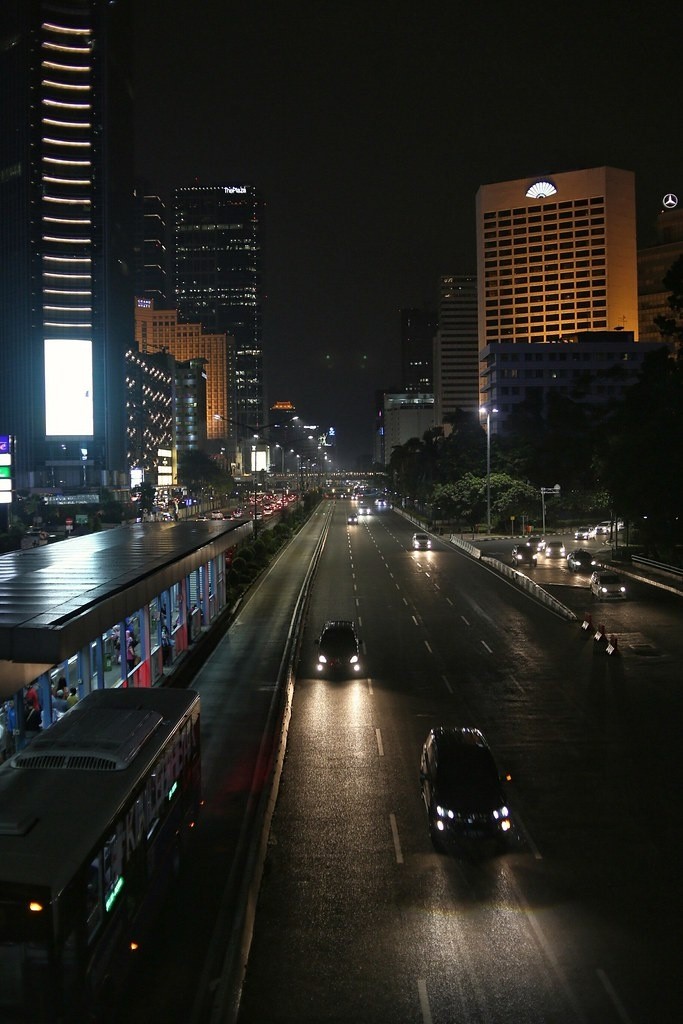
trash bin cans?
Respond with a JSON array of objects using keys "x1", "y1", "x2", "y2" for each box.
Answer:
[
  {"x1": 104, "y1": 653, "x2": 113, "y2": 671},
  {"x1": 439, "y1": 526, "x2": 443, "y2": 535}
]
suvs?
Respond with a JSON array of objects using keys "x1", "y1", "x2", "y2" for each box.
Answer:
[
  {"x1": 419, "y1": 724, "x2": 514, "y2": 854},
  {"x1": 512, "y1": 542, "x2": 537, "y2": 569},
  {"x1": 313, "y1": 620, "x2": 365, "y2": 680}
]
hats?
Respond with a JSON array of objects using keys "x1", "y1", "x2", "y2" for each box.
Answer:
[
  {"x1": 128, "y1": 637, "x2": 133, "y2": 643},
  {"x1": 57, "y1": 689, "x2": 64, "y2": 697}
]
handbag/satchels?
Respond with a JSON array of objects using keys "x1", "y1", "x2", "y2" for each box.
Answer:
[
  {"x1": 167, "y1": 637, "x2": 176, "y2": 647},
  {"x1": 133, "y1": 657, "x2": 141, "y2": 666},
  {"x1": 24, "y1": 731, "x2": 37, "y2": 746}
]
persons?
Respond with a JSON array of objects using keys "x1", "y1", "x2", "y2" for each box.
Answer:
[
  {"x1": 110, "y1": 617, "x2": 139, "y2": 672},
  {"x1": 7, "y1": 676, "x2": 79, "y2": 748},
  {"x1": 150, "y1": 599, "x2": 204, "y2": 642}
]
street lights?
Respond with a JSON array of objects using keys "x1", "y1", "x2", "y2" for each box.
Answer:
[{"x1": 481, "y1": 404, "x2": 498, "y2": 535}]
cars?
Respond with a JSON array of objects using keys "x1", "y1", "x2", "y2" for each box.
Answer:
[
  {"x1": 525, "y1": 518, "x2": 624, "y2": 561},
  {"x1": 161, "y1": 489, "x2": 298, "y2": 522},
  {"x1": 325, "y1": 486, "x2": 390, "y2": 525},
  {"x1": 413, "y1": 532, "x2": 432, "y2": 551},
  {"x1": 566, "y1": 549, "x2": 594, "y2": 573},
  {"x1": 591, "y1": 570, "x2": 627, "y2": 603}
]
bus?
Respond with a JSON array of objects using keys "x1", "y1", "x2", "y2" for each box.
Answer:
[{"x1": 0, "y1": 687, "x2": 203, "y2": 1022}]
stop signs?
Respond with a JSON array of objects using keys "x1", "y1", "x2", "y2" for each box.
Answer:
[{"x1": 65, "y1": 516, "x2": 73, "y2": 526}]
